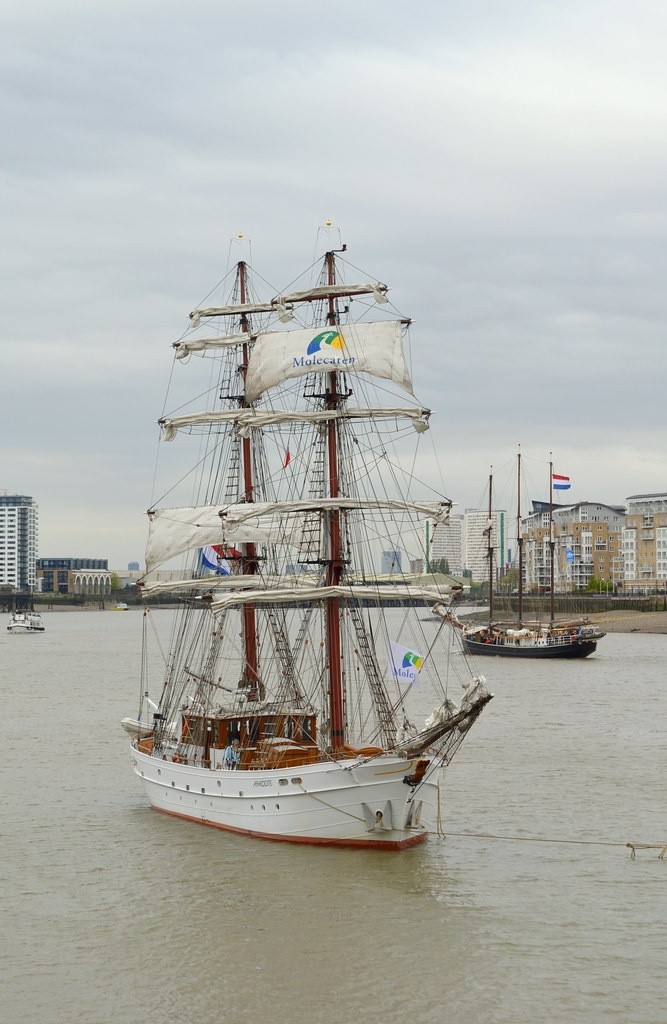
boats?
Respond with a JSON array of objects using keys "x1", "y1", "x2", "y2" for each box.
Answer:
[
  {"x1": 6, "y1": 607, "x2": 46, "y2": 635},
  {"x1": 110, "y1": 600, "x2": 130, "y2": 612}
]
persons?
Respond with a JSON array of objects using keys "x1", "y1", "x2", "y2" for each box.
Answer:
[
  {"x1": 480, "y1": 632, "x2": 509, "y2": 645},
  {"x1": 537, "y1": 626, "x2": 582, "y2": 646},
  {"x1": 223, "y1": 739, "x2": 242, "y2": 770},
  {"x1": 466, "y1": 633, "x2": 474, "y2": 641}
]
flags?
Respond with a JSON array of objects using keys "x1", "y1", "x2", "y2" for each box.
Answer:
[
  {"x1": 202, "y1": 544, "x2": 242, "y2": 576},
  {"x1": 552, "y1": 473, "x2": 571, "y2": 490},
  {"x1": 387, "y1": 640, "x2": 426, "y2": 688}
]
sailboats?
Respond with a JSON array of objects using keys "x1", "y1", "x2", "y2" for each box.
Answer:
[
  {"x1": 117, "y1": 215, "x2": 498, "y2": 851},
  {"x1": 459, "y1": 441, "x2": 608, "y2": 660}
]
